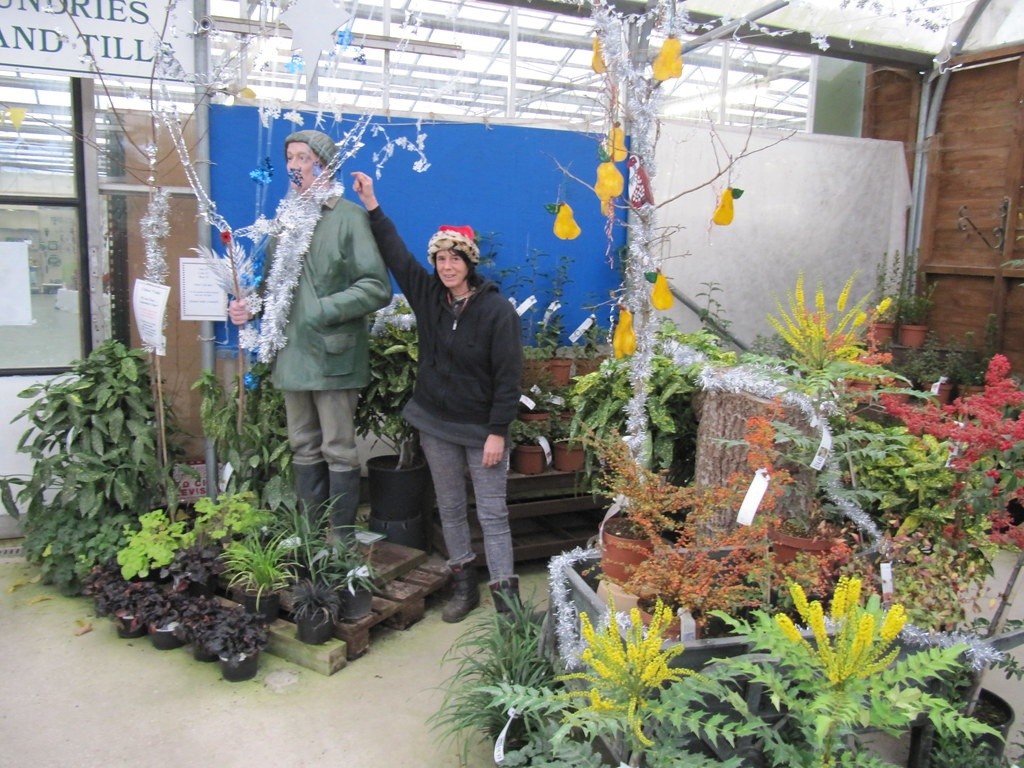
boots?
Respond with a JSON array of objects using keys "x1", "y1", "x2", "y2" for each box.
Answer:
[
  {"x1": 442, "y1": 555, "x2": 480, "y2": 622},
  {"x1": 490, "y1": 575, "x2": 543, "y2": 642}
]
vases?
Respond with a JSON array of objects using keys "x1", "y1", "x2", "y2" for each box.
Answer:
[{"x1": 366, "y1": 456, "x2": 427, "y2": 554}]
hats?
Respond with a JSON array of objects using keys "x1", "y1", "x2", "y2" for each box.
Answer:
[{"x1": 427, "y1": 224, "x2": 479, "y2": 269}]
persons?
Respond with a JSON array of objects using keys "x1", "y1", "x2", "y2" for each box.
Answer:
[
  {"x1": 350, "y1": 171, "x2": 548, "y2": 644},
  {"x1": 228, "y1": 129, "x2": 393, "y2": 569}
]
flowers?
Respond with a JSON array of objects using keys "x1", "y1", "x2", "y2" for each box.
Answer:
[{"x1": 356, "y1": 294, "x2": 423, "y2": 468}]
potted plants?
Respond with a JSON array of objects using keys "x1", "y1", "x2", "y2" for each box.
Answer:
[
  {"x1": 428, "y1": 243, "x2": 1024, "y2": 767},
  {"x1": 78, "y1": 486, "x2": 386, "y2": 682}
]
